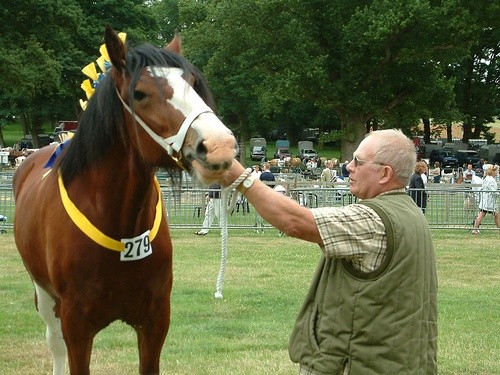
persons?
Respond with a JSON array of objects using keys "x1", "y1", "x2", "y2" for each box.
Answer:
[
  {"x1": 220, "y1": 129, "x2": 436, "y2": 375},
  {"x1": 251, "y1": 162, "x2": 275, "y2": 188},
  {"x1": 195, "y1": 182, "x2": 221, "y2": 235},
  {"x1": 303, "y1": 155, "x2": 349, "y2": 200},
  {"x1": 431, "y1": 161, "x2": 440, "y2": 183},
  {"x1": 463, "y1": 159, "x2": 500, "y2": 235},
  {"x1": 16, "y1": 139, "x2": 33, "y2": 151},
  {"x1": 408, "y1": 160, "x2": 429, "y2": 213}
]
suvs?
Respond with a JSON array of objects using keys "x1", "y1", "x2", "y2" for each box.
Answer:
[{"x1": 19, "y1": 133, "x2": 54, "y2": 149}]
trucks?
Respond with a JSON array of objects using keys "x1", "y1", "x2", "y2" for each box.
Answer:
[
  {"x1": 250, "y1": 138, "x2": 268, "y2": 161},
  {"x1": 274, "y1": 140, "x2": 293, "y2": 160},
  {"x1": 297, "y1": 140, "x2": 319, "y2": 163},
  {"x1": 412, "y1": 136, "x2": 488, "y2": 174}
]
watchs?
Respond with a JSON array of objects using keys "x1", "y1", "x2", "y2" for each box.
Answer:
[{"x1": 239, "y1": 174, "x2": 256, "y2": 195}]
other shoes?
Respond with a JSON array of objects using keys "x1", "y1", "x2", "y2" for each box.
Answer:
[{"x1": 195, "y1": 230, "x2": 208, "y2": 235}]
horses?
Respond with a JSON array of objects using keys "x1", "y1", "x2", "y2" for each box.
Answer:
[{"x1": 12, "y1": 24, "x2": 241, "y2": 375}]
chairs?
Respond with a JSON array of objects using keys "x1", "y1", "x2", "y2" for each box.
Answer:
[
  {"x1": 193, "y1": 193, "x2": 208, "y2": 218},
  {"x1": 231, "y1": 194, "x2": 250, "y2": 215}
]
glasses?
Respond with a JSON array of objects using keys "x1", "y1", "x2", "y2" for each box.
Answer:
[{"x1": 352, "y1": 152, "x2": 386, "y2": 167}]
